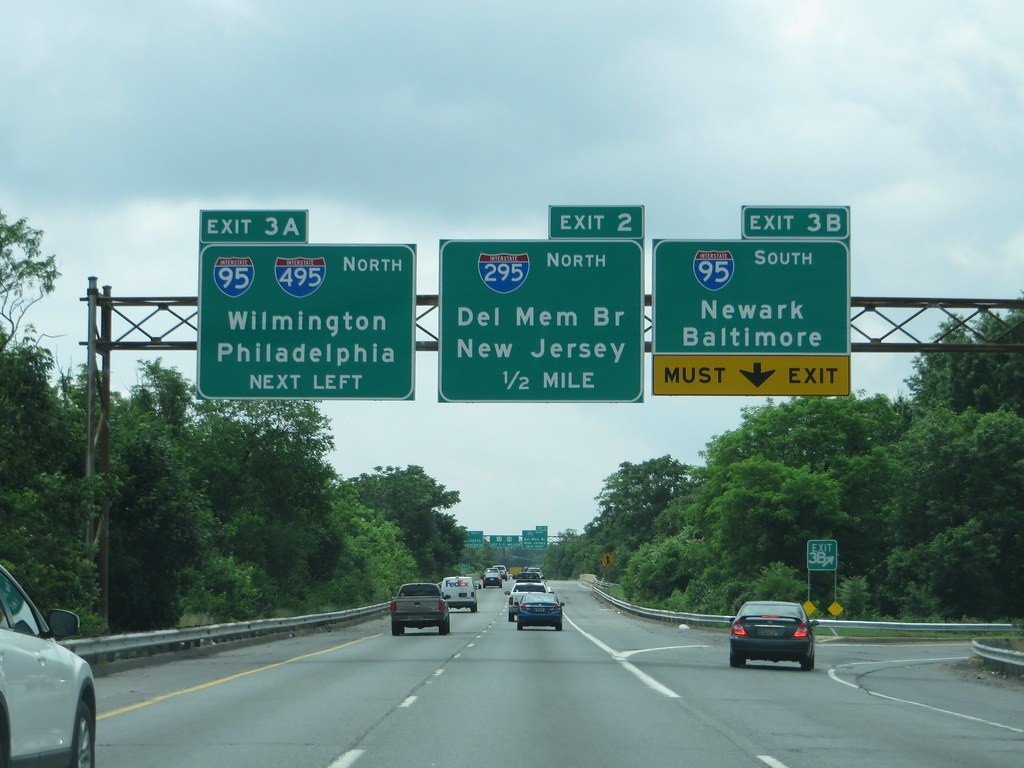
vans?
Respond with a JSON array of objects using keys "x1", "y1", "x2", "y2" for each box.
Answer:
[{"x1": 440, "y1": 576, "x2": 482, "y2": 613}]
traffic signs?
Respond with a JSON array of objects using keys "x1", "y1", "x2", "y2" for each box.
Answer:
[
  {"x1": 196, "y1": 210, "x2": 418, "y2": 402},
  {"x1": 435, "y1": 204, "x2": 645, "y2": 405},
  {"x1": 807, "y1": 540, "x2": 837, "y2": 572},
  {"x1": 650, "y1": 207, "x2": 852, "y2": 397}
]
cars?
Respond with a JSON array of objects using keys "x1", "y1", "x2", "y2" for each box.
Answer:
[
  {"x1": 1, "y1": 563, "x2": 97, "y2": 768},
  {"x1": 479, "y1": 564, "x2": 553, "y2": 622},
  {"x1": 727, "y1": 599, "x2": 816, "y2": 672},
  {"x1": 513, "y1": 592, "x2": 566, "y2": 631}
]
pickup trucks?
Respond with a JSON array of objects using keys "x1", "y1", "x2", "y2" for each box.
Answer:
[{"x1": 390, "y1": 582, "x2": 452, "y2": 636}]
